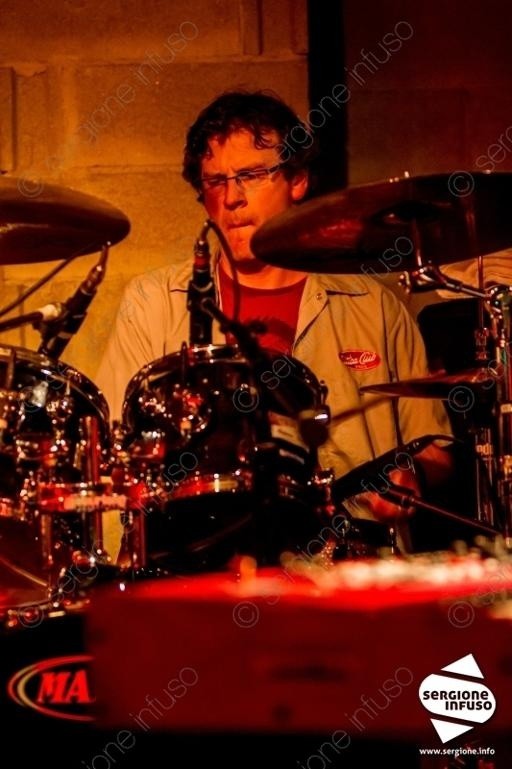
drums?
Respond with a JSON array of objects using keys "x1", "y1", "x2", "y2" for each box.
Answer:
[
  {"x1": 0, "y1": 344, "x2": 111, "y2": 538},
  {"x1": 116, "y1": 338, "x2": 330, "y2": 552},
  {"x1": 0, "y1": 615, "x2": 101, "y2": 769}
]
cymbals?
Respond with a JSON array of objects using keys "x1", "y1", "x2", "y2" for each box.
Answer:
[
  {"x1": 355, "y1": 370, "x2": 511, "y2": 403},
  {"x1": 248, "y1": 163, "x2": 512, "y2": 276},
  {"x1": 0, "y1": 172, "x2": 130, "y2": 262}
]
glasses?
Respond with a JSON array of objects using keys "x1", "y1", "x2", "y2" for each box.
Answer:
[{"x1": 197, "y1": 161, "x2": 285, "y2": 196}]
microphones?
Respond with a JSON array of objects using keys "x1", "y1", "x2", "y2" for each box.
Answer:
[
  {"x1": 186, "y1": 238, "x2": 216, "y2": 346},
  {"x1": 38, "y1": 260, "x2": 105, "y2": 361},
  {"x1": 334, "y1": 434, "x2": 434, "y2": 502}
]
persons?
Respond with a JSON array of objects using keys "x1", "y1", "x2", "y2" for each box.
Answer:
[{"x1": 93, "y1": 92, "x2": 456, "y2": 557}]
